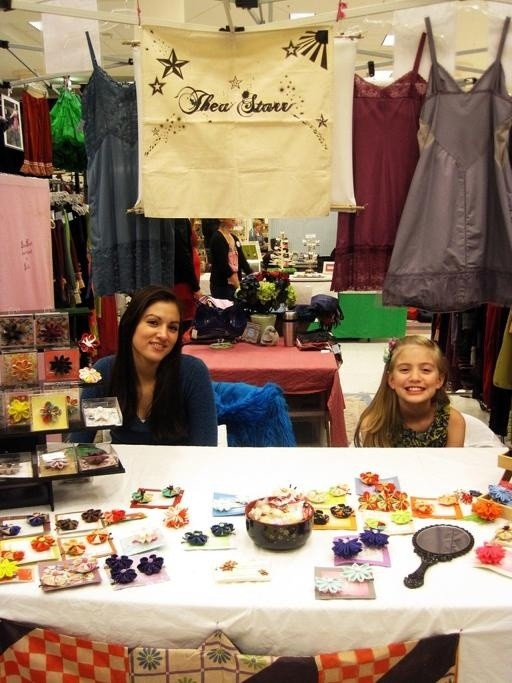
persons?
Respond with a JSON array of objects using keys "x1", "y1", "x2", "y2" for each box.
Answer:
[
  {"x1": 68, "y1": 286, "x2": 219, "y2": 445},
  {"x1": 249, "y1": 218, "x2": 268, "y2": 251},
  {"x1": 353, "y1": 335, "x2": 466, "y2": 447},
  {"x1": 209, "y1": 217, "x2": 254, "y2": 300}
]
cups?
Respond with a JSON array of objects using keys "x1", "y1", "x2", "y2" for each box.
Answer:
[{"x1": 283, "y1": 311, "x2": 298, "y2": 346}]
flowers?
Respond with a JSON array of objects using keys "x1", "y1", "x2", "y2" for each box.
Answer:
[{"x1": 233, "y1": 250, "x2": 297, "y2": 316}]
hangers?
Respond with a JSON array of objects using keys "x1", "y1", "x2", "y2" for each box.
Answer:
[{"x1": 49, "y1": 190, "x2": 90, "y2": 230}]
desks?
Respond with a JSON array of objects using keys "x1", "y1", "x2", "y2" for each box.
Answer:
[{"x1": 0, "y1": 272, "x2": 511, "y2": 683}]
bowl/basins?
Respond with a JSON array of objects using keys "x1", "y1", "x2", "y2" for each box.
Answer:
[{"x1": 245, "y1": 497, "x2": 314, "y2": 551}]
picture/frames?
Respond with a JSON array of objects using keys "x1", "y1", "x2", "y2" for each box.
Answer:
[{"x1": 1, "y1": 94, "x2": 24, "y2": 153}]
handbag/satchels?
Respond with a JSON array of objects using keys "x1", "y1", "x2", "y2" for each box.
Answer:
[{"x1": 190, "y1": 297, "x2": 247, "y2": 340}]
